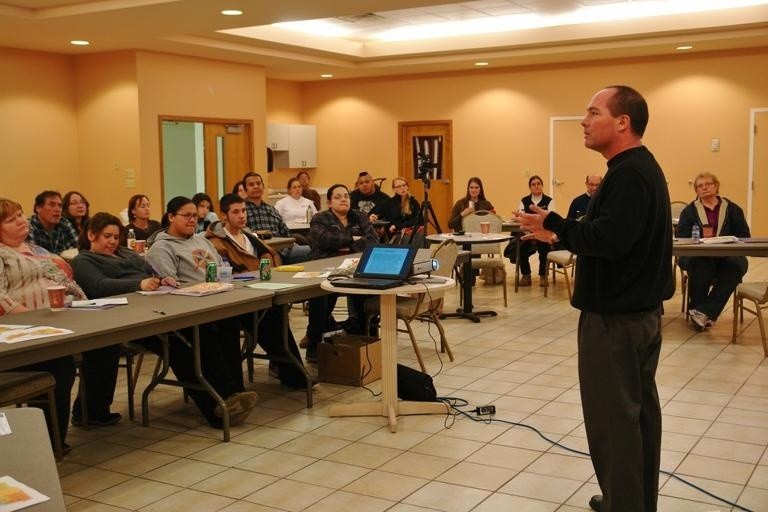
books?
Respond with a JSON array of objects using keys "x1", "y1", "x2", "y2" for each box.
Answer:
[
  {"x1": 142, "y1": 281, "x2": 238, "y2": 298},
  {"x1": 699, "y1": 236, "x2": 768, "y2": 245}
]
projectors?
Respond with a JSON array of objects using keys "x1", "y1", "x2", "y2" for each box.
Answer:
[{"x1": 409, "y1": 259, "x2": 439, "y2": 276}]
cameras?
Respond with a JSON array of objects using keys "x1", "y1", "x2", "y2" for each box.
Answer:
[{"x1": 418, "y1": 151, "x2": 438, "y2": 181}]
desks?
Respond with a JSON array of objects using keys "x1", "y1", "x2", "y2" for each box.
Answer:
[
  {"x1": 235, "y1": 246, "x2": 472, "y2": 410},
  {"x1": 59, "y1": 246, "x2": 145, "y2": 263},
  {"x1": 1, "y1": 405, "x2": 67, "y2": 512},
  {"x1": 257, "y1": 238, "x2": 296, "y2": 251},
  {"x1": 500, "y1": 219, "x2": 557, "y2": 293},
  {"x1": 1, "y1": 279, "x2": 276, "y2": 442},
  {"x1": 424, "y1": 231, "x2": 517, "y2": 323},
  {"x1": 318, "y1": 273, "x2": 456, "y2": 433},
  {"x1": 285, "y1": 221, "x2": 389, "y2": 233},
  {"x1": 671, "y1": 236, "x2": 768, "y2": 258}
]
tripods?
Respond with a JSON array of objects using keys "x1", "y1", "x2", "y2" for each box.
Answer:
[{"x1": 408, "y1": 184, "x2": 463, "y2": 285}]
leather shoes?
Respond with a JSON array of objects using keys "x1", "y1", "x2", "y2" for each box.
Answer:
[{"x1": 590, "y1": 494, "x2": 603, "y2": 509}]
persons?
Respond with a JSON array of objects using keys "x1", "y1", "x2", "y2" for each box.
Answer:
[
  {"x1": 123, "y1": 194, "x2": 160, "y2": 245},
  {"x1": 508, "y1": 84, "x2": 677, "y2": 511},
  {"x1": 448, "y1": 177, "x2": 496, "y2": 285},
  {"x1": 205, "y1": 193, "x2": 318, "y2": 391},
  {"x1": 193, "y1": 169, "x2": 311, "y2": 259},
  {"x1": 298, "y1": 172, "x2": 321, "y2": 213},
  {"x1": 567, "y1": 174, "x2": 602, "y2": 223},
  {"x1": 519, "y1": 176, "x2": 558, "y2": 287},
  {"x1": 349, "y1": 172, "x2": 426, "y2": 247},
  {"x1": 144, "y1": 196, "x2": 246, "y2": 396},
  {"x1": 27, "y1": 191, "x2": 93, "y2": 260},
  {"x1": 0, "y1": 198, "x2": 123, "y2": 458},
  {"x1": 674, "y1": 172, "x2": 753, "y2": 334},
  {"x1": 298, "y1": 180, "x2": 381, "y2": 351},
  {"x1": 72, "y1": 210, "x2": 260, "y2": 430}
]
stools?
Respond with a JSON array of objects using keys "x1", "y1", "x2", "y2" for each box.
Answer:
[
  {"x1": 129, "y1": 336, "x2": 194, "y2": 403},
  {"x1": 70, "y1": 340, "x2": 148, "y2": 432},
  {"x1": 0, "y1": 369, "x2": 65, "y2": 463}
]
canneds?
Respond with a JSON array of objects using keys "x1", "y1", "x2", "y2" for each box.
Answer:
[
  {"x1": 206, "y1": 262, "x2": 217, "y2": 282},
  {"x1": 260, "y1": 259, "x2": 271, "y2": 280}
]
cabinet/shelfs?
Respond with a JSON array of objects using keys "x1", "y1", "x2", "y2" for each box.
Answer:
[
  {"x1": 275, "y1": 125, "x2": 318, "y2": 168},
  {"x1": 265, "y1": 123, "x2": 288, "y2": 151}
]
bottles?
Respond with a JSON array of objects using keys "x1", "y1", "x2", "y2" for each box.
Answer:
[
  {"x1": 220, "y1": 259, "x2": 232, "y2": 284},
  {"x1": 126, "y1": 227, "x2": 136, "y2": 248},
  {"x1": 691, "y1": 222, "x2": 700, "y2": 243}
]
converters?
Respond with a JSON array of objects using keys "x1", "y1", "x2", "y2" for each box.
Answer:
[{"x1": 477, "y1": 406, "x2": 495, "y2": 415}]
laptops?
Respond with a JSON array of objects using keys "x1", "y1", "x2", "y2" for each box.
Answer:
[{"x1": 330, "y1": 243, "x2": 420, "y2": 289}]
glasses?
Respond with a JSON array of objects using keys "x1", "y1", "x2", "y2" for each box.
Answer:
[{"x1": 177, "y1": 212, "x2": 198, "y2": 219}]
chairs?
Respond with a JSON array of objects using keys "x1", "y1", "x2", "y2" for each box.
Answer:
[
  {"x1": 672, "y1": 222, "x2": 745, "y2": 325},
  {"x1": 361, "y1": 238, "x2": 458, "y2": 372},
  {"x1": 732, "y1": 281, "x2": 768, "y2": 356},
  {"x1": 544, "y1": 250, "x2": 577, "y2": 301},
  {"x1": 668, "y1": 201, "x2": 696, "y2": 293},
  {"x1": 461, "y1": 209, "x2": 509, "y2": 307}
]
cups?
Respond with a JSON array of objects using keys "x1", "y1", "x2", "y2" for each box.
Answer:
[
  {"x1": 479, "y1": 221, "x2": 490, "y2": 236},
  {"x1": 133, "y1": 239, "x2": 145, "y2": 253},
  {"x1": 468, "y1": 199, "x2": 475, "y2": 209},
  {"x1": 46, "y1": 285, "x2": 67, "y2": 312}
]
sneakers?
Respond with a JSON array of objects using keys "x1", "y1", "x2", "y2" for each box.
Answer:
[
  {"x1": 540, "y1": 275, "x2": 549, "y2": 285},
  {"x1": 215, "y1": 392, "x2": 257, "y2": 425},
  {"x1": 72, "y1": 413, "x2": 121, "y2": 426},
  {"x1": 688, "y1": 309, "x2": 712, "y2": 331},
  {"x1": 518, "y1": 274, "x2": 531, "y2": 285},
  {"x1": 300, "y1": 335, "x2": 318, "y2": 362}
]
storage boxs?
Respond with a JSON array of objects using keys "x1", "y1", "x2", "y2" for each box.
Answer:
[{"x1": 317, "y1": 335, "x2": 382, "y2": 387}]
